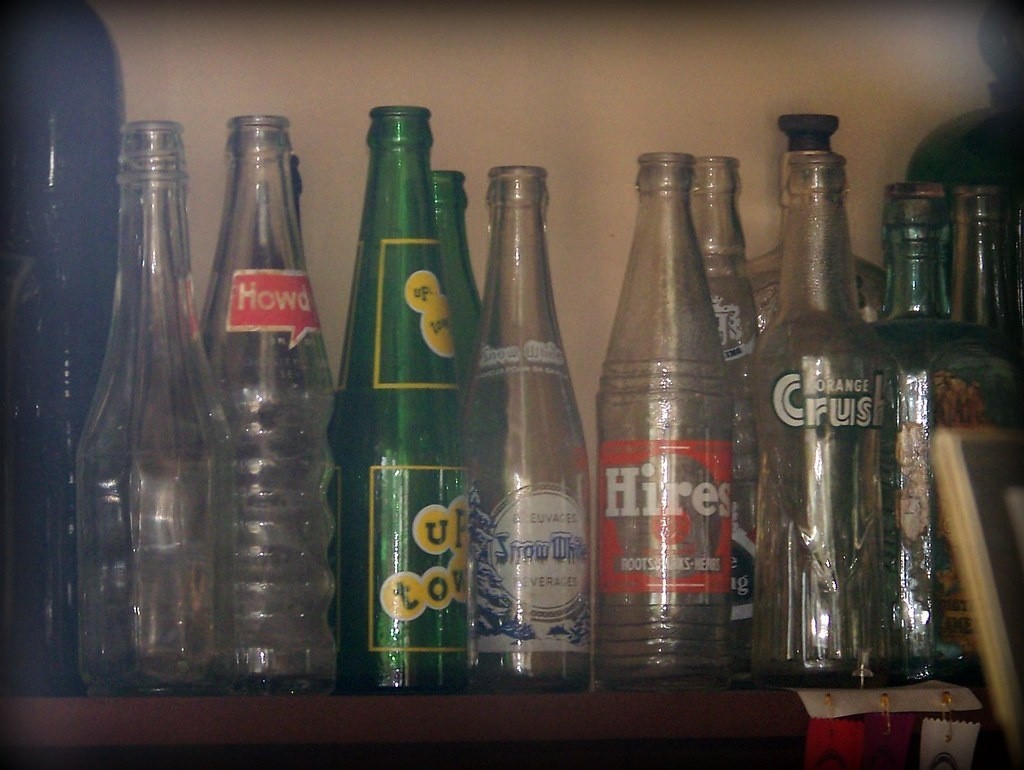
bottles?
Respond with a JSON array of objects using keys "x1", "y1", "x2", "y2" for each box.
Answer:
[
  {"x1": 197, "y1": 111, "x2": 342, "y2": 695},
  {"x1": 454, "y1": 165, "x2": 594, "y2": 693},
  {"x1": 75, "y1": 120, "x2": 232, "y2": 695},
  {"x1": 743, "y1": 114, "x2": 889, "y2": 332},
  {"x1": 323, "y1": 106, "x2": 486, "y2": 701},
  {"x1": 907, "y1": 0, "x2": 1024, "y2": 190},
  {"x1": 752, "y1": 151, "x2": 898, "y2": 693},
  {"x1": 592, "y1": 153, "x2": 736, "y2": 691},
  {"x1": 0, "y1": 0, "x2": 124, "y2": 699},
  {"x1": 687, "y1": 157, "x2": 761, "y2": 663},
  {"x1": 866, "y1": 177, "x2": 1024, "y2": 684}
]
